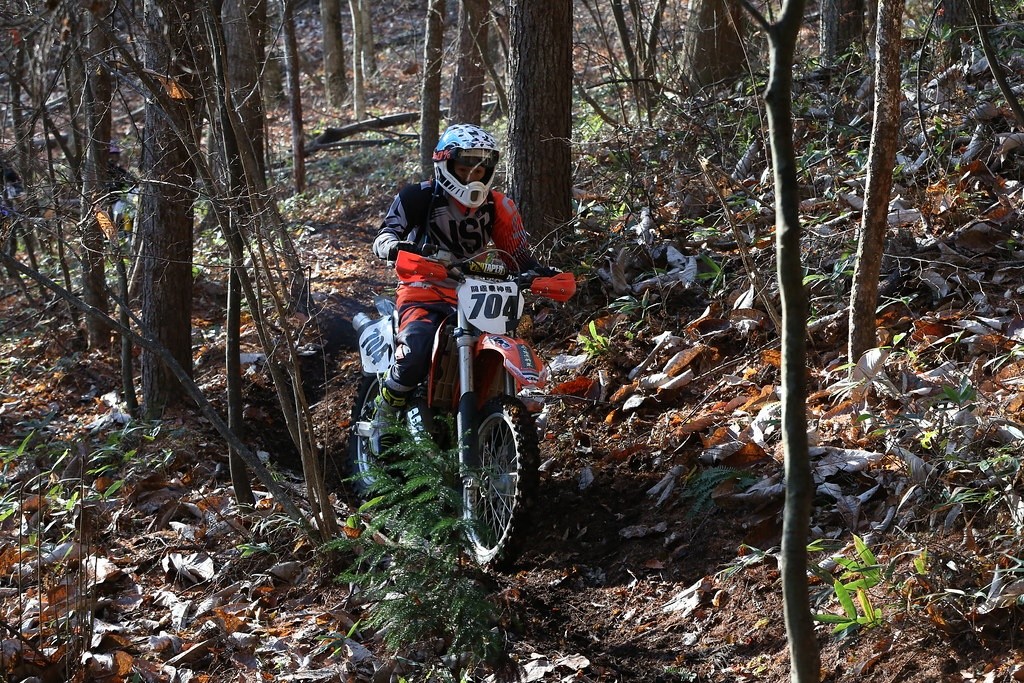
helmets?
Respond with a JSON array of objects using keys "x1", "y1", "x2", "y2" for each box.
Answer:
[{"x1": 433, "y1": 121, "x2": 501, "y2": 209}]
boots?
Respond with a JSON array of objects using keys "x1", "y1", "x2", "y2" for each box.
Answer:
[{"x1": 370, "y1": 369, "x2": 418, "y2": 461}]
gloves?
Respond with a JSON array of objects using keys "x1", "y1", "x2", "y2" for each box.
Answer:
[
  {"x1": 522, "y1": 265, "x2": 564, "y2": 284},
  {"x1": 376, "y1": 240, "x2": 420, "y2": 262}
]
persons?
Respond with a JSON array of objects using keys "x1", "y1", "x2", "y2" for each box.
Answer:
[{"x1": 370, "y1": 123, "x2": 563, "y2": 460}]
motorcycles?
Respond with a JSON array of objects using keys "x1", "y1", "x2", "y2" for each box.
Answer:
[{"x1": 342, "y1": 242, "x2": 554, "y2": 573}]
